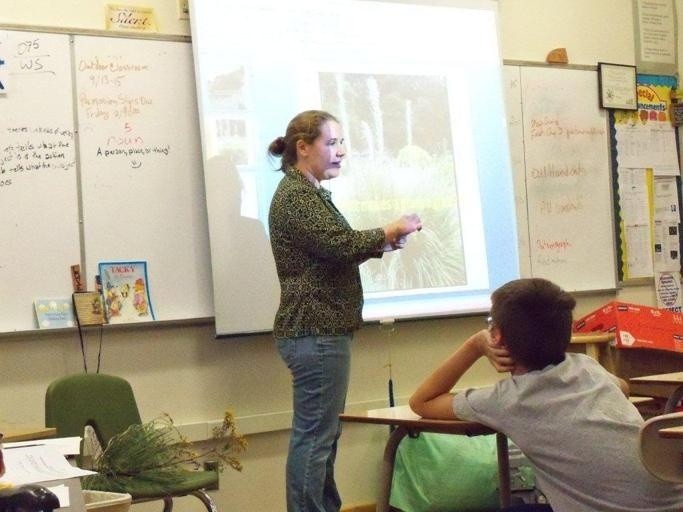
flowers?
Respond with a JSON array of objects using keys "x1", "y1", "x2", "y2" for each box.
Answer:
[{"x1": 74, "y1": 409, "x2": 249, "y2": 489}]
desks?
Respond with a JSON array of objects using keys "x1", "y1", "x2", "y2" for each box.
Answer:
[{"x1": 333, "y1": 394, "x2": 659, "y2": 508}]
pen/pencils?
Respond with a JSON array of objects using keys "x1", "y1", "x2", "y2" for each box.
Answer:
[{"x1": 4, "y1": 442, "x2": 45, "y2": 450}]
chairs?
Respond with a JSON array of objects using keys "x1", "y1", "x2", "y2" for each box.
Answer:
[
  {"x1": 43, "y1": 373, "x2": 222, "y2": 512},
  {"x1": 636, "y1": 411, "x2": 681, "y2": 486}
]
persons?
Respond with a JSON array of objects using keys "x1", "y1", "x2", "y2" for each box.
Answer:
[
  {"x1": 268, "y1": 110, "x2": 422, "y2": 511},
  {"x1": 407, "y1": 276, "x2": 682, "y2": 511}
]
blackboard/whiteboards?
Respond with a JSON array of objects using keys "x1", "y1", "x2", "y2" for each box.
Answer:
[{"x1": 0, "y1": 21, "x2": 622, "y2": 342}]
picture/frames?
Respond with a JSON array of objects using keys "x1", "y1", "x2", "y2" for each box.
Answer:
[{"x1": 598, "y1": 61, "x2": 641, "y2": 111}]
